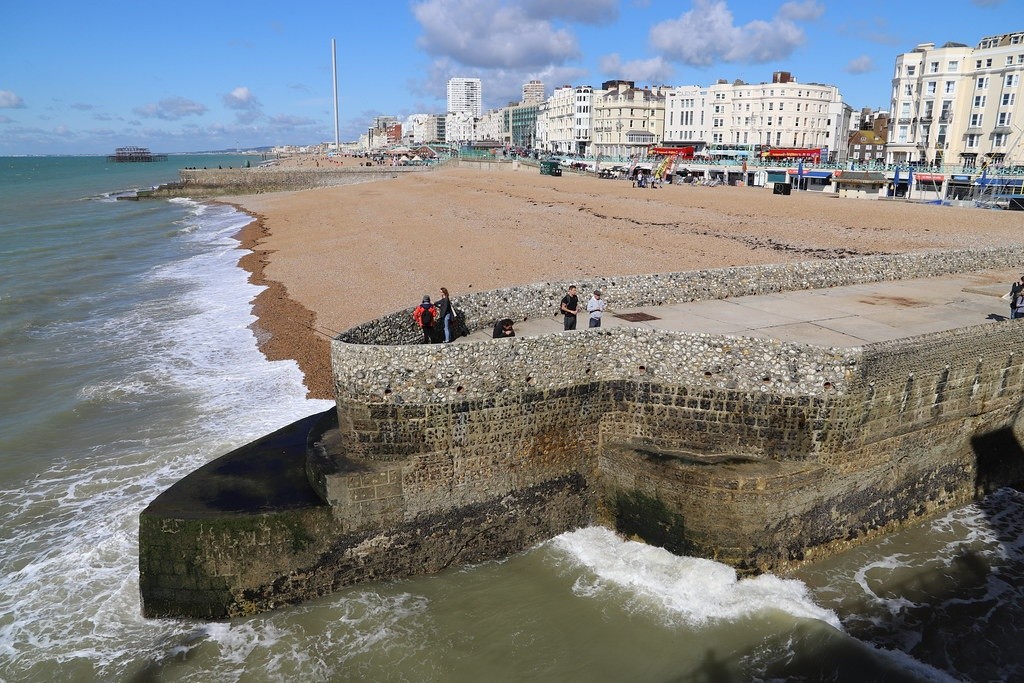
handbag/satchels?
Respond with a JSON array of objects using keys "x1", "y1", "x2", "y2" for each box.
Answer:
[
  {"x1": 450, "y1": 304, "x2": 457, "y2": 318},
  {"x1": 1001, "y1": 282, "x2": 1018, "y2": 303}
]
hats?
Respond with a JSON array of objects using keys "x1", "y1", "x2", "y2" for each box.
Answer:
[
  {"x1": 423, "y1": 295, "x2": 430, "y2": 301},
  {"x1": 594, "y1": 290, "x2": 601, "y2": 296}
]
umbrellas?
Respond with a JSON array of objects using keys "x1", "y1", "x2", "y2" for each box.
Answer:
[
  {"x1": 979, "y1": 169, "x2": 986, "y2": 189},
  {"x1": 893, "y1": 166, "x2": 913, "y2": 200}
]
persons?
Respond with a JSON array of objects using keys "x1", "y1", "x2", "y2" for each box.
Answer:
[
  {"x1": 1010, "y1": 276, "x2": 1024, "y2": 319},
  {"x1": 434, "y1": 288, "x2": 456, "y2": 343},
  {"x1": 414, "y1": 295, "x2": 436, "y2": 344},
  {"x1": 1016, "y1": 288, "x2": 1024, "y2": 318},
  {"x1": 560, "y1": 286, "x2": 579, "y2": 330},
  {"x1": 493, "y1": 319, "x2": 515, "y2": 338},
  {"x1": 587, "y1": 291, "x2": 604, "y2": 328},
  {"x1": 637, "y1": 172, "x2": 663, "y2": 188}
]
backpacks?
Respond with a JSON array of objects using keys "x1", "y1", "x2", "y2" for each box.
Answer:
[
  {"x1": 560, "y1": 296, "x2": 570, "y2": 314},
  {"x1": 420, "y1": 303, "x2": 433, "y2": 326}
]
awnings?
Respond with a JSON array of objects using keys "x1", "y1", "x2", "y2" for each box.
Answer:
[
  {"x1": 802, "y1": 171, "x2": 832, "y2": 178},
  {"x1": 975, "y1": 178, "x2": 1023, "y2": 187}
]
